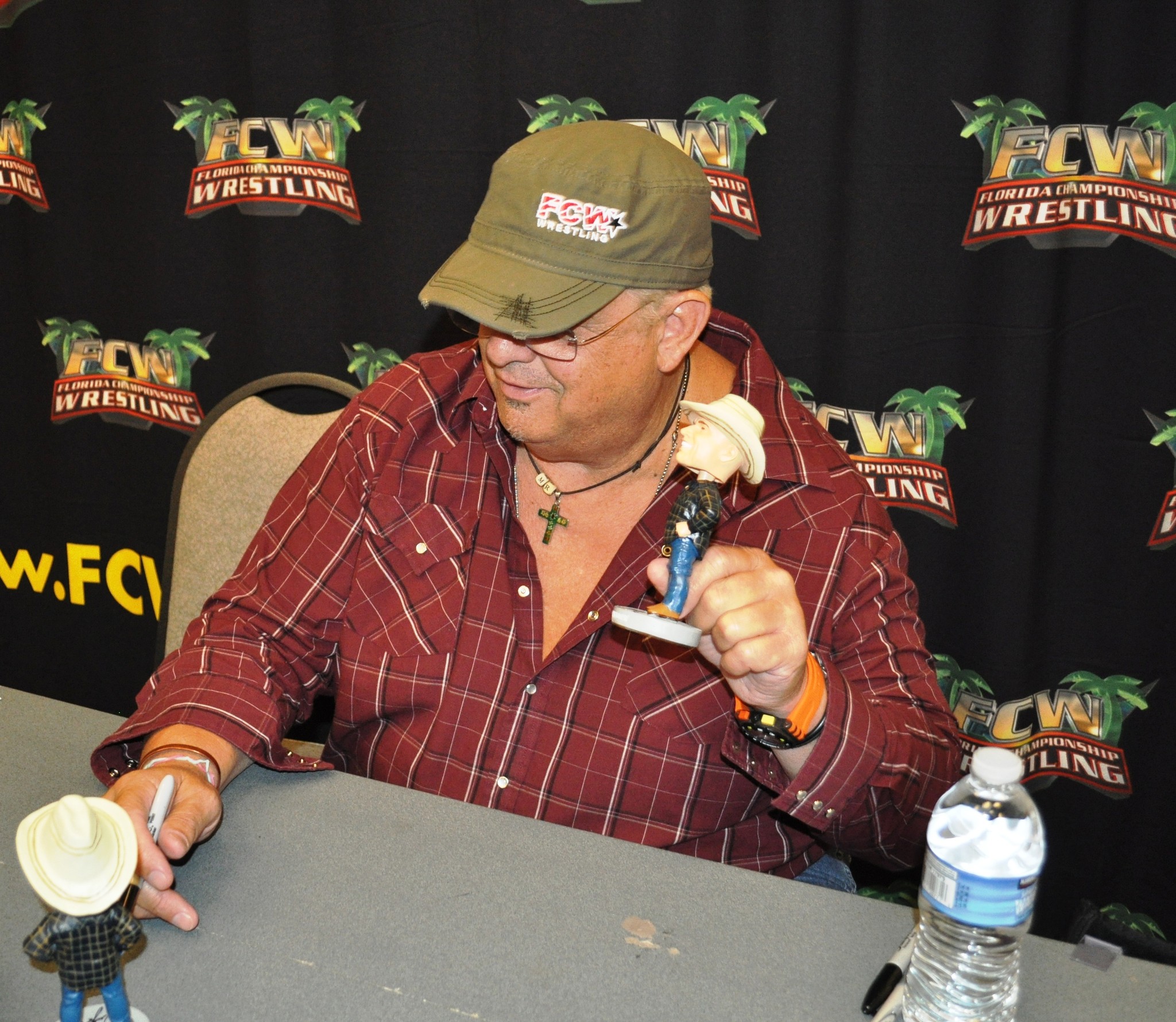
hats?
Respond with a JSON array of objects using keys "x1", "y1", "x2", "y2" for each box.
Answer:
[{"x1": 418, "y1": 121, "x2": 714, "y2": 340}]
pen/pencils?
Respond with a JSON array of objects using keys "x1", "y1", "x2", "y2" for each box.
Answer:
[
  {"x1": 871, "y1": 965, "x2": 911, "y2": 1022},
  {"x1": 120, "y1": 774, "x2": 175, "y2": 913}
]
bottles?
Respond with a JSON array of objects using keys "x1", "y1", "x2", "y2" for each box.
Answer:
[{"x1": 902, "y1": 746, "x2": 1046, "y2": 1022}]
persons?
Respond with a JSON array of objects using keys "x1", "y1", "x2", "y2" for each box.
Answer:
[
  {"x1": 16, "y1": 792, "x2": 143, "y2": 1022},
  {"x1": 87, "y1": 122, "x2": 965, "y2": 928},
  {"x1": 639, "y1": 391, "x2": 768, "y2": 622}
]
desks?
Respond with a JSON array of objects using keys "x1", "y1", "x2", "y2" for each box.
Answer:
[{"x1": 1, "y1": 686, "x2": 1176, "y2": 1022}]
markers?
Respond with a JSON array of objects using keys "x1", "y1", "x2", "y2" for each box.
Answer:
[{"x1": 862, "y1": 922, "x2": 921, "y2": 1013}]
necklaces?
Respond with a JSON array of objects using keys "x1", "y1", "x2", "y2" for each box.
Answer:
[{"x1": 522, "y1": 341, "x2": 693, "y2": 549}]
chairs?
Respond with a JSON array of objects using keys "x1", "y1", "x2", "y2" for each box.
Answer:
[{"x1": 154, "y1": 373, "x2": 362, "y2": 760}]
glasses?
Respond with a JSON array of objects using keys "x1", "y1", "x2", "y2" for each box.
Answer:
[{"x1": 446, "y1": 297, "x2": 658, "y2": 362}]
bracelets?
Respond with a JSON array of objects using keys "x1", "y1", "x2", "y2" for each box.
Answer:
[{"x1": 134, "y1": 739, "x2": 225, "y2": 794}]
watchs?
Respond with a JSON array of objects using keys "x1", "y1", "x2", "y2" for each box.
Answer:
[{"x1": 732, "y1": 652, "x2": 822, "y2": 748}]
